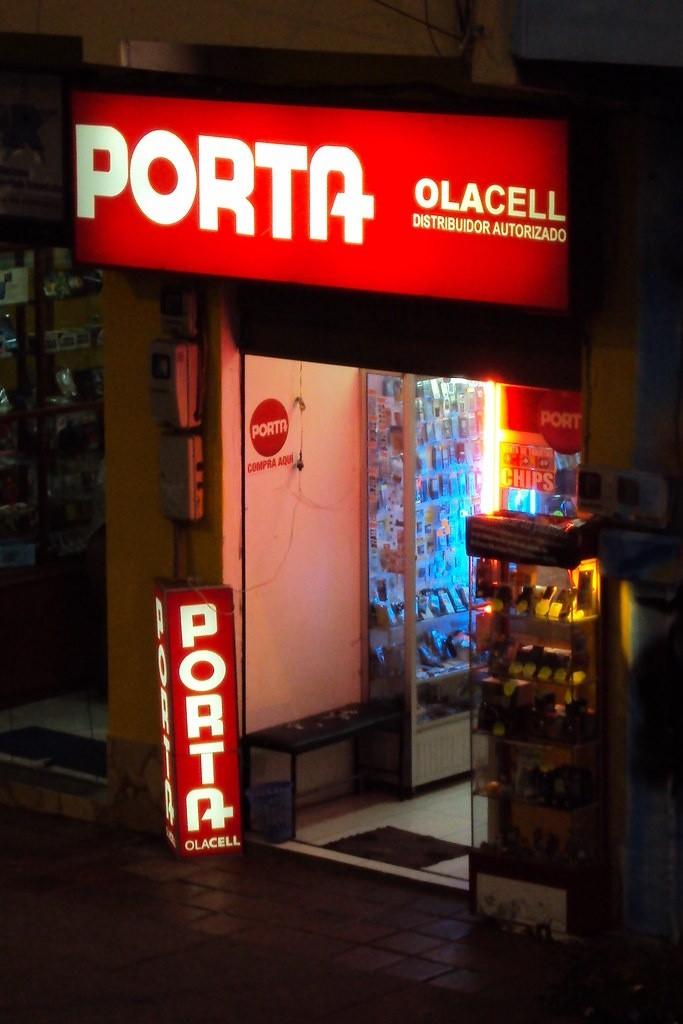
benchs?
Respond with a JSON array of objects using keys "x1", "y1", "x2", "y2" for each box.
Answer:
[{"x1": 242, "y1": 702, "x2": 404, "y2": 839}]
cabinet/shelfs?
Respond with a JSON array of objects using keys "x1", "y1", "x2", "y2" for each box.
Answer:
[
  {"x1": 0, "y1": 239, "x2": 107, "y2": 710},
  {"x1": 358, "y1": 368, "x2": 489, "y2": 799},
  {"x1": 466, "y1": 508, "x2": 620, "y2": 937}
]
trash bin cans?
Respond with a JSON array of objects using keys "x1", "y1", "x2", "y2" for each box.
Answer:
[{"x1": 245, "y1": 780, "x2": 293, "y2": 844}]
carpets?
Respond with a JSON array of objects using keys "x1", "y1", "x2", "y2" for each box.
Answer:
[
  {"x1": 321, "y1": 824, "x2": 469, "y2": 869},
  {"x1": 0, "y1": 725, "x2": 107, "y2": 778}
]
